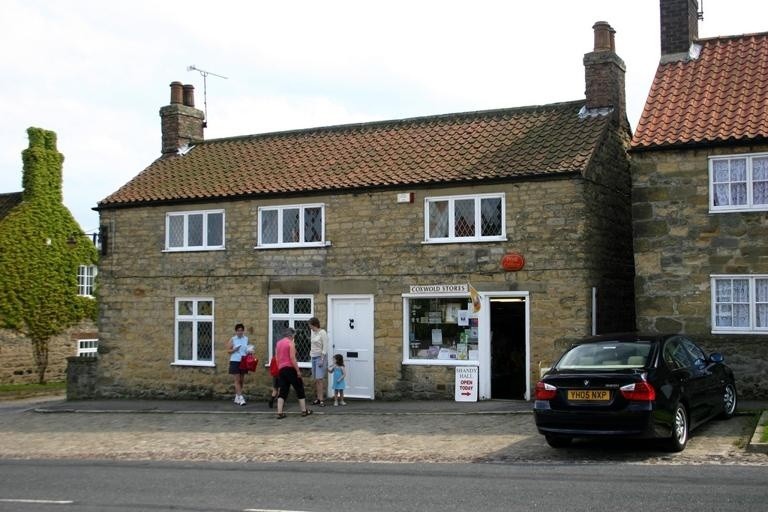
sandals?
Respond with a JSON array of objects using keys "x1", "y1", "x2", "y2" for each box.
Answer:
[
  {"x1": 276, "y1": 412, "x2": 287, "y2": 419},
  {"x1": 301, "y1": 408, "x2": 313, "y2": 417},
  {"x1": 310, "y1": 399, "x2": 320, "y2": 405},
  {"x1": 320, "y1": 400, "x2": 326, "y2": 407}
]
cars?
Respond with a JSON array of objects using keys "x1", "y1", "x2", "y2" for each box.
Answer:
[{"x1": 531, "y1": 333, "x2": 738, "y2": 453}]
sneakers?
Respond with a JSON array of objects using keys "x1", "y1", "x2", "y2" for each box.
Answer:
[
  {"x1": 239, "y1": 399, "x2": 246, "y2": 405},
  {"x1": 334, "y1": 399, "x2": 338, "y2": 407},
  {"x1": 233, "y1": 398, "x2": 240, "y2": 404},
  {"x1": 269, "y1": 399, "x2": 274, "y2": 408},
  {"x1": 339, "y1": 400, "x2": 346, "y2": 405}
]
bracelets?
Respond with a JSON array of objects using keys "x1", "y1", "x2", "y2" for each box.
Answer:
[{"x1": 320, "y1": 358, "x2": 324, "y2": 361}]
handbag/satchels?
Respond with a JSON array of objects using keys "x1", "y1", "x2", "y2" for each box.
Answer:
[{"x1": 239, "y1": 352, "x2": 259, "y2": 372}]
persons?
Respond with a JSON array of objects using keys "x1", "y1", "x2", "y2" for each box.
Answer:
[
  {"x1": 274, "y1": 327, "x2": 314, "y2": 419},
  {"x1": 307, "y1": 317, "x2": 328, "y2": 409},
  {"x1": 325, "y1": 354, "x2": 347, "y2": 407},
  {"x1": 225, "y1": 322, "x2": 256, "y2": 408},
  {"x1": 268, "y1": 354, "x2": 280, "y2": 409}
]
